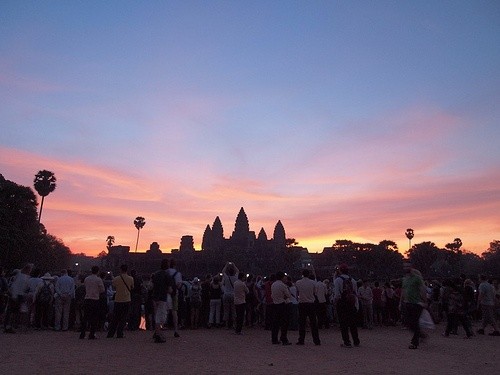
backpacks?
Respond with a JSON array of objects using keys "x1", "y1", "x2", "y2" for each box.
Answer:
[
  {"x1": 338, "y1": 275, "x2": 353, "y2": 299},
  {"x1": 35, "y1": 280, "x2": 52, "y2": 308}
]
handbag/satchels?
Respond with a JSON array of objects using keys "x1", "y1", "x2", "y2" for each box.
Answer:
[
  {"x1": 418, "y1": 309, "x2": 436, "y2": 336},
  {"x1": 385, "y1": 297, "x2": 392, "y2": 309}
]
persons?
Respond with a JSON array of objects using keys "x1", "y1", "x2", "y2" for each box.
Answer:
[
  {"x1": 399, "y1": 261, "x2": 427, "y2": 349},
  {"x1": 0, "y1": 258, "x2": 500, "y2": 347}
]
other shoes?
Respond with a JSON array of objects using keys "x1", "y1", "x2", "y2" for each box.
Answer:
[
  {"x1": 450, "y1": 332, "x2": 457, "y2": 336},
  {"x1": 89, "y1": 336, "x2": 95, "y2": 339},
  {"x1": 464, "y1": 336, "x2": 472, "y2": 339},
  {"x1": 174, "y1": 332, "x2": 179, "y2": 337},
  {"x1": 4, "y1": 328, "x2": 16, "y2": 334},
  {"x1": 107, "y1": 335, "x2": 113, "y2": 338},
  {"x1": 409, "y1": 343, "x2": 417, "y2": 349},
  {"x1": 272, "y1": 342, "x2": 281, "y2": 344},
  {"x1": 155, "y1": 335, "x2": 162, "y2": 343},
  {"x1": 80, "y1": 334, "x2": 85, "y2": 339},
  {"x1": 153, "y1": 332, "x2": 156, "y2": 338},
  {"x1": 355, "y1": 340, "x2": 360, "y2": 346},
  {"x1": 488, "y1": 331, "x2": 500, "y2": 336},
  {"x1": 401, "y1": 326, "x2": 407, "y2": 329},
  {"x1": 283, "y1": 342, "x2": 292, "y2": 345},
  {"x1": 319, "y1": 321, "x2": 406, "y2": 328},
  {"x1": 116, "y1": 336, "x2": 123, "y2": 338},
  {"x1": 22, "y1": 326, "x2": 80, "y2": 332},
  {"x1": 161, "y1": 325, "x2": 206, "y2": 330},
  {"x1": 340, "y1": 343, "x2": 352, "y2": 348},
  {"x1": 216, "y1": 324, "x2": 220, "y2": 328},
  {"x1": 296, "y1": 342, "x2": 304, "y2": 345},
  {"x1": 208, "y1": 323, "x2": 211, "y2": 328},
  {"x1": 470, "y1": 318, "x2": 483, "y2": 325},
  {"x1": 477, "y1": 329, "x2": 484, "y2": 335}
]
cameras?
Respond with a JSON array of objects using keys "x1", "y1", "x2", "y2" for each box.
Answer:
[
  {"x1": 220, "y1": 273, "x2": 223, "y2": 276},
  {"x1": 284, "y1": 273, "x2": 288, "y2": 275},
  {"x1": 308, "y1": 264, "x2": 311, "y2": 266},
  {"x1": 229, "y1": 262, "x2": 232, "y2": 265},
  {"x1": 247, "y1": 274, "x2": 249, "y2": 277},
  {"x1": 76, "y1": 263, "x2": 78, "y2": 266},
  {"x1": 108, "y1": 272, "x2": 110, "y2": 274}
]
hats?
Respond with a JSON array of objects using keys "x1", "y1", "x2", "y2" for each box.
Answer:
[
  {"x1": 336, "y1": 264, "x2": 348, "y2": 271},
  {"x1": 40, "y1": 272, "x2": 54, "y2": 279}
]
